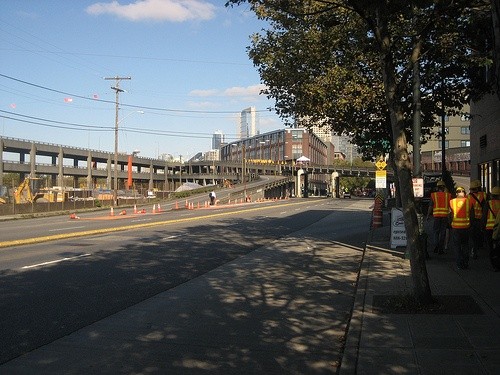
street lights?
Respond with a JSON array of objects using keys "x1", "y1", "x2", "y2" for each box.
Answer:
[{"x1": 114, "y1": 110, "x2": 144, "y2": 207}]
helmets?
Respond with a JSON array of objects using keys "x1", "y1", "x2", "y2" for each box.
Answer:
[
  {"x1": 490, "y1": 186, "x2": 500, "y2": 195},
  {"x1": 437, "y1": 180, "x2": 445, "y2": 185},
  {"x1": 469, "y1": 180, "x2": 481, "y2": 189},
  {"x1": 456, "y1": 187, "x2": 464, "y2": 191}
]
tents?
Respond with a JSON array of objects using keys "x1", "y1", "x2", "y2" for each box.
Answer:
[{"x1": 296, "y1": 156, "x2": 310, "y2": 166}]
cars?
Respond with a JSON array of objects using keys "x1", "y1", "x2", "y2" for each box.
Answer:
[{"x1": 344, "y1": 192, "x2": 351, "y2": 199}]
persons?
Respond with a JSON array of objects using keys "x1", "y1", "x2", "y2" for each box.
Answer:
[
  {"x1": 286, "y1": 188, "x2": 290, "y2": 198},
  {"x1": 388, "y1": 188, "x2": 392, "y2": 198},
  {"x1": 209, "y1": 189, "x2": 216, "y2": 205},
  {"x1": 426, "y1": 181, "x2": 451, "y2": 254},
  {"x1": 449, "y1": 180, "x2": 500, "y2": 271}
]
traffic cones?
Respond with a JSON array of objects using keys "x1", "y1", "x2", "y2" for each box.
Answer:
[
  {"x1": 71, "y1": 213, "x2": 79, "y2": 218},
  {"x1": 138, "y1": 209, "x2": 146, "y2": 214},
  {"x1": 120, "y1": 210, "x2": 126, "y2": 215}
]
